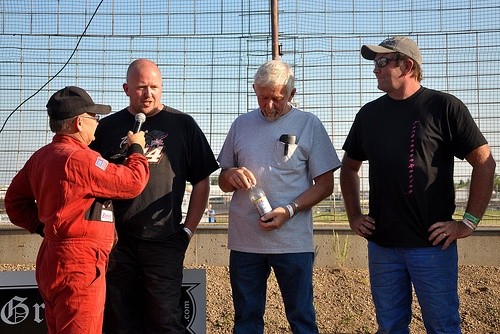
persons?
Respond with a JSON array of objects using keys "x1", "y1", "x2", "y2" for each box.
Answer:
[
  {"x1": 340, "y1": 37, "x2": 496, "y2": 334},
  {"x1": 88, "y1": 59, "x2": 219, "y2": 334},
  {"x1": 216, "y1": 59, "x2": 343, "y2": 334},
  {"x1": 209, "y1": 204, "x2": 215, "y2": 222},
  {"x1": 4, "y1": 85, "x2": 150, "y2": 334}
]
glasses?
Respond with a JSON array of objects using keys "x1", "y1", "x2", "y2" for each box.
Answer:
[
  {"x1": 374, "y1": 57, "x2": 409, "y2": 68},
  {"x1": 72, "y1": 115, "x2": 100, "y2": 123}
]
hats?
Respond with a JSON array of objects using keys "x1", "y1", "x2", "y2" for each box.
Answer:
[
  {"x1": 361, "y1": 36, "x2": 422, "y2": 66},
  {"x1": 46, "y1": 86, "x2": 112, "y2": 121}
]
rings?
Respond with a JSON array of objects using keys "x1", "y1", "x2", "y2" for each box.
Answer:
[{"x1": 444, "y1": 233, "x2": 447, "y2": 236}]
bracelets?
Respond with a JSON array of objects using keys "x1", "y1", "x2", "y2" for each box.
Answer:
[
  {"x1": 184, "y1": 226, "x2": 194, "y2": 238},
  {"x1": 293, "y1": 202, "x2": 299, "y2": 214},
  {"x1": 286, "y1": 204, "x2": 294, "y2": 217},
  {"x1": 126, "y1": 143, "x2": 143, "y2": 155},
  {"x1": 462, "y1": 212, "x2": 480, "y2": 231}
]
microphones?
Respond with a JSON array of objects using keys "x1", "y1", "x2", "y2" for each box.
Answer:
[{"x1": 133, "y1": 112, "x2": 146, "y2": 134}]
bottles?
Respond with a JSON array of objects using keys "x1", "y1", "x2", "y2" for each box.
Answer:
[
  {"x1": 248, "y1": 184, "x2": 274, "y2": 223},
  {"x1": 209, "y1": 209, "x2": 215, "y2": 223}
]
